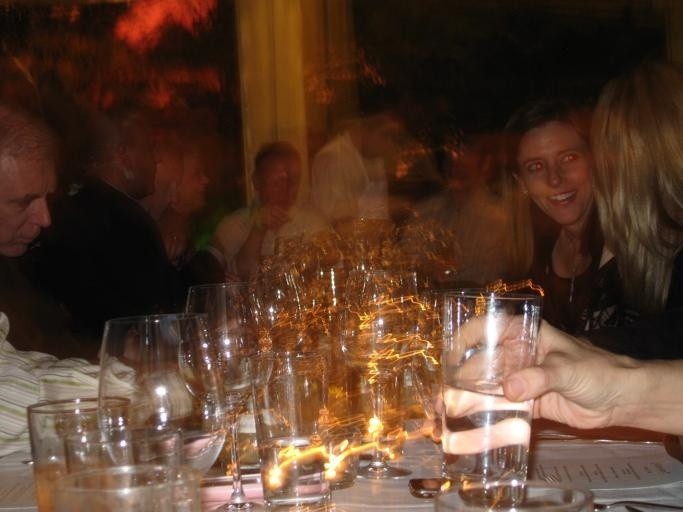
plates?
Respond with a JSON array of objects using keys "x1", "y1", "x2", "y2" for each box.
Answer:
[{"x1": 436, "y1": 482, "x2": 594, "y2": 511}]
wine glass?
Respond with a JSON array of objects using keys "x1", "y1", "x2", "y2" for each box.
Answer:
[
  {"x1": 177, "y1": 280, "x2": 274, "y2": 512},
  {"x1": 226, "y1": 219, "x2": 439, "y2": 480}
]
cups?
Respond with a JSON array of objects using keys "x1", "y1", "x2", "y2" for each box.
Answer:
[
  {"x1": 439, "y1": 292, "x2": 543, "y2": 508},
  {"x1": 27, "y1": 314, "x2": 227, "y2": 510},
  {"x1": 248, "y1": 349, "x2": 335, "y2": 512}
]
